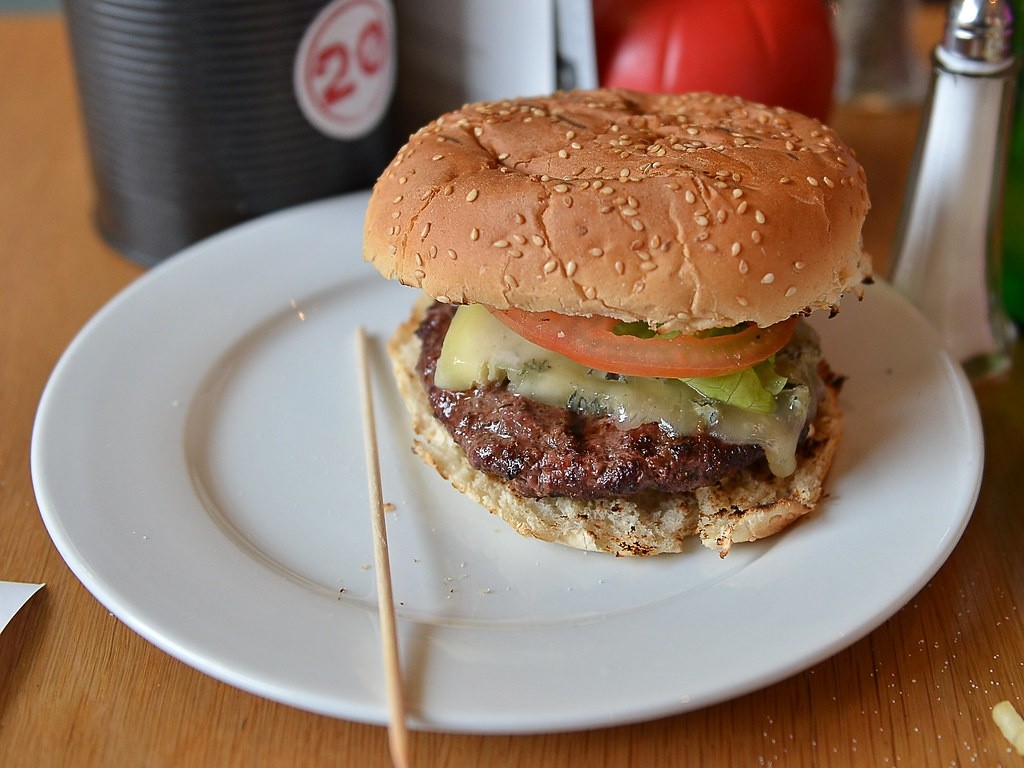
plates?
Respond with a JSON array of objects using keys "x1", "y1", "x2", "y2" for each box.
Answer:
[{"x1": 26, "y1": 189, "x2": 987, "y2": 737}]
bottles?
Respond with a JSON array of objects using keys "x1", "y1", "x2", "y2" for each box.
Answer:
[{"x1": 894, "y1": 0, "x2": 1018, "y2": 383}]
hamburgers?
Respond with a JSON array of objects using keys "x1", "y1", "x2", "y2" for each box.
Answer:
[{"x1": 359, "y1": 83, "x2": 872, "y2": 563}]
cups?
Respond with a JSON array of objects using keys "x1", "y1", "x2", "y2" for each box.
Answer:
[{"x1": 62, "y1": 0, "x2": 402, "y2": 278}]
